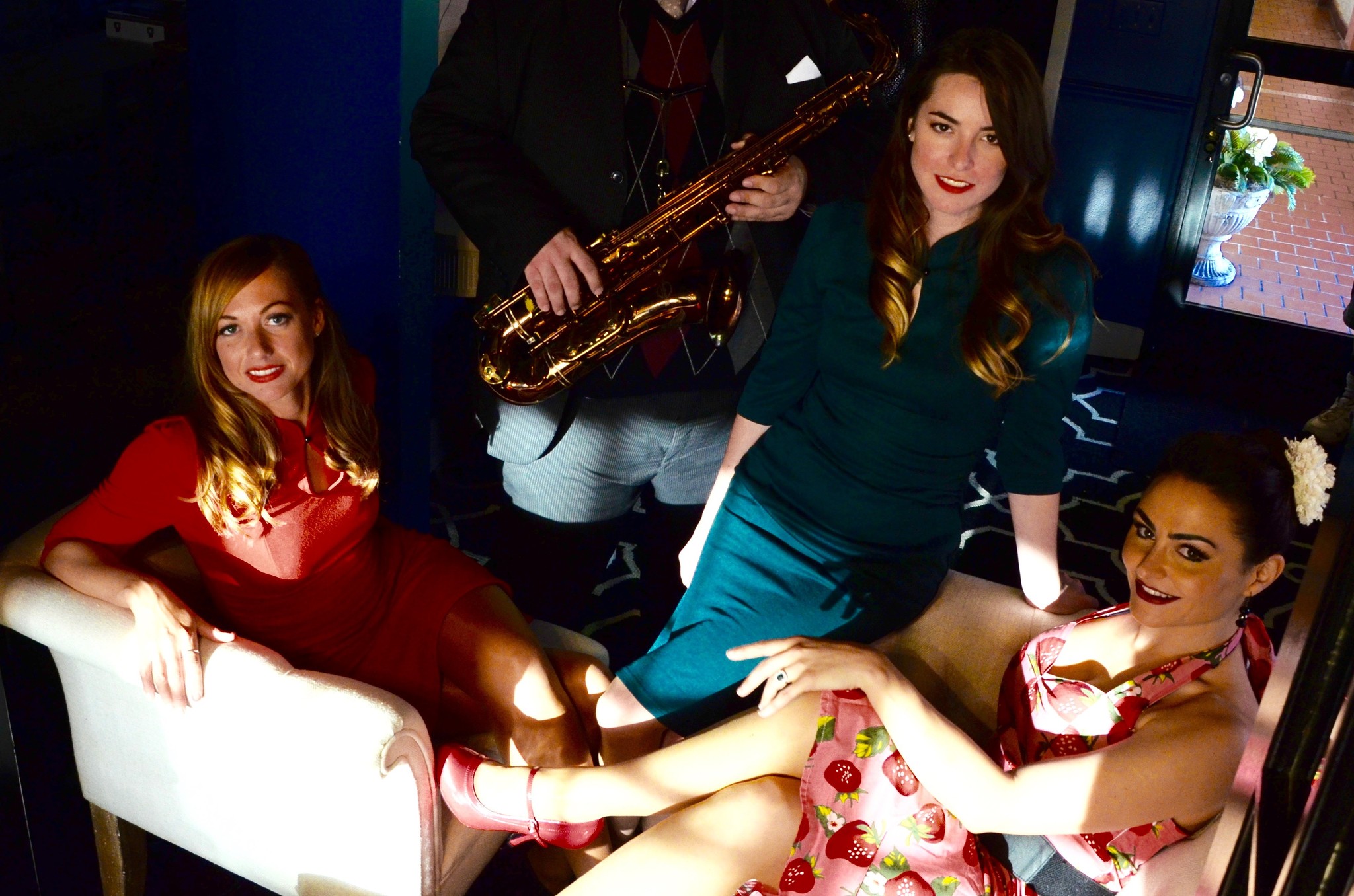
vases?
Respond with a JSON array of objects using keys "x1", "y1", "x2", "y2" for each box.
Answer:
[{"x1": 1190, "y1": 186, "x2": 1271, "y2": 287}]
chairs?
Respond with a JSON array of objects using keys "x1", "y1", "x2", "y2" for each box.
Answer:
[
  {"x1": 0, "y1": 506, "x2": 608, "y2": 896},
  {"x1": 857, "y1": 568, "x2": 1221, "y2": 896}
]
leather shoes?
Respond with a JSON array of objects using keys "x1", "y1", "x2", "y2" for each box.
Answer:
[{"x1": 435, "y1": 743, "x2": 604, "y2": 850}]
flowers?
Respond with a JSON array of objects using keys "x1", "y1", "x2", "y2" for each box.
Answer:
[
  {"x1": 1216, "y1": 83, "x2": 1315, "y2": 213},
  {"x1": 1284, "y1": 436, "x2": 1338, "y2": 525}
]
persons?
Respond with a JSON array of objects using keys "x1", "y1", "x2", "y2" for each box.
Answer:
[
  {"x1": 409, "y1": 0, "x2": 924, "y2": 686},
  {"x1": 44, "y1": 231, "x2": 622, "y2": 895},
  {"x1": 594, "y1": 28, "x2": 1102, "y2": 846},
  {"x1": 433, "y1": 430, "x2": 1339, "y2": 896}
]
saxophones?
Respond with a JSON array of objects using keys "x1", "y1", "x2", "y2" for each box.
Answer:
[{"x1": 472, "y1": 0, "x2": 906, "y2": 406}]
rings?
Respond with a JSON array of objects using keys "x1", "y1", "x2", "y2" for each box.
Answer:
[
  {"x1": 773, "y1": 668, "x2": 790, "y2": 685},
  {"x1": 180, "y1": 648, "x2": 201, "y2": 659}
]
